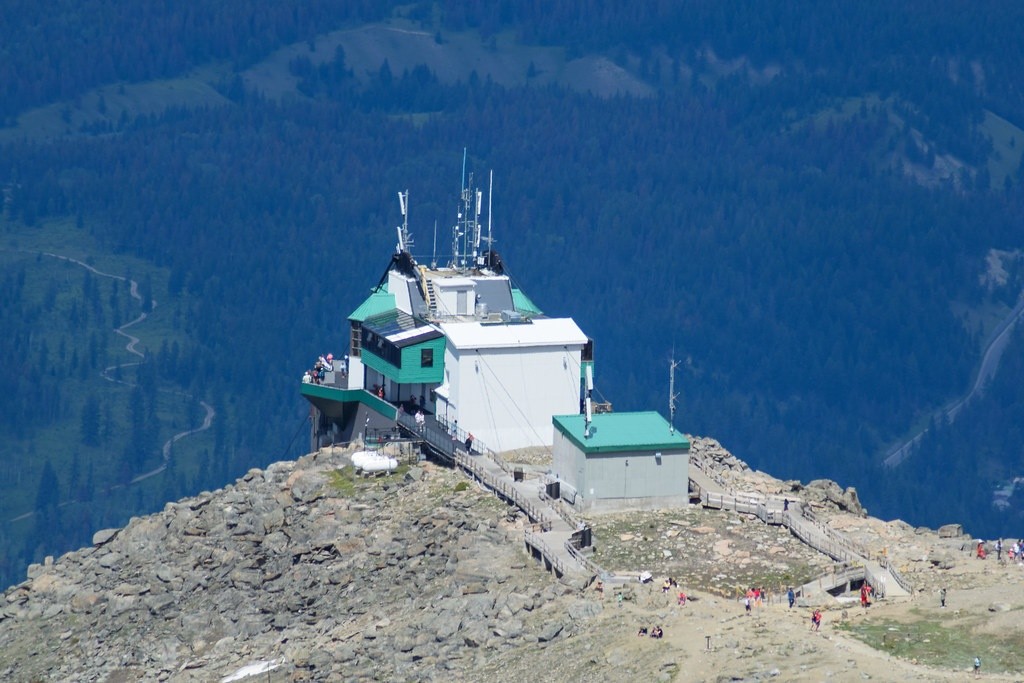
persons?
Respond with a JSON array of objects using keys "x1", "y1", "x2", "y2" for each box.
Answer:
[
  {"x1": 996, "y1": 538, "x2": 1024, "y2": 561},
  {"x1": 744, "y1": 586, "x2": 766, "y2": 612},
  {"x1": 662, "y1": 578, "x2": 677, "y2": 593},
  {"x1": 940, "y1": 589, "x2": 946, "y2": 606},
  {"x1": 978, "y1": 543, "x2": 986, "y2": 559},
  {"x1": 595, "y1": 581, "x2": 602, "y2": 593},
  {"x1": 400, "y1": 396, "x2": 416, "y2": 413},
  {"x1": 974, "y1": 656, "x2": 981, "y2": 675},
  {"x1": 618, "y1": 592, "x2": 622, "y2": 608},
  {"x1": 303, "y1": 353, "x2": 349, "y2": 384},
  {"x1": 809, "y1": 609, "x2": 822, "y2": 632},
  {"x1": 465, "y1": 433, "x2": 473, "y2": 455},
  {"x1": 415, "y1": 411, "x2": 426, "y2": 432},
  {"x1": 639, "y1": 626, "x2": 662, "y2": 638},
  {"x1": 859, "y1": 583, "x2": 873, "y2": 607},
  {"x1": 577, "y1": 520, "x2": 585, "y2": 530},
  {"x1": 788, "y1": 588, "x2": 794, "y2": 608},
  {"x1": 452, "y1": 430, "x2": 457, "y2": 450},
  {"x1": 378, "y1": 388, "x2": 383, "y2": 398}
]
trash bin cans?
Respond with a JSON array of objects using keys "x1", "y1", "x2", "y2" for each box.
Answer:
[
  {"x1": 513, "y1": 466, "x2": 523, "y2": 481},
  {"x1": 584, "y1": 528, "x2": 591, "y2": 546},
  {"x1": 545, "y1": 481, "x2": 560, "y2": 499}
]
